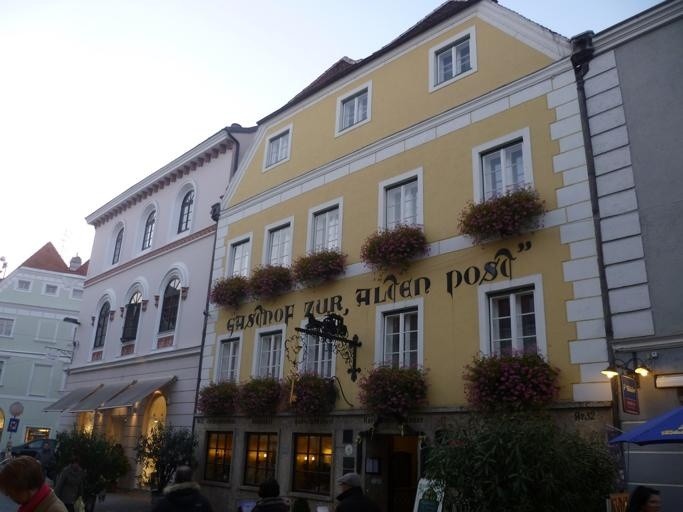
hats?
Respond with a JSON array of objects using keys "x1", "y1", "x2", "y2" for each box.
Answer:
[
  {"x1": 169, "y1": 465, "x2": 200, "y2": 482},
  {"x1": 262, "y1": 477, "x2": 279, "y2": 496},
  {"x1": 337, "y1": 473, "x2": 361, "y2": 487}
]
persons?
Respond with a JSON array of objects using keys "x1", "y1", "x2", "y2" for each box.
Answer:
[
  {"x1": 4, "y1": 439, "x2": 101, "y2": 512},
  {"x1": 626, "y1": 483, "x2": 664, "y2": 512},
  {"x1": 0, "y1": 454, "x2": 72, "y2": 512},
  {"x1": 332, "y1": 472, "x2": 379, "y2": 511},
  {"x1": 250, "y1": 476, "x2": 291, "y2": 512},
  {"x1": 152, "y1": 465, "x2": 212, "y2": 510}
]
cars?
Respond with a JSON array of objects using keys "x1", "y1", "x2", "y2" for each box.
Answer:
[{"x1": 0, "y1": 437, "x2": 62, "y2": 465}]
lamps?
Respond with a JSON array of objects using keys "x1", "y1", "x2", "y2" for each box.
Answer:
[{"x1": 600, "y1": 358, "x2": 651, "y2": 380}]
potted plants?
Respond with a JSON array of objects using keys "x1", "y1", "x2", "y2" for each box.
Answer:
[{"x1": 94, "y1": 442, "x2": 130, "y2": 493}]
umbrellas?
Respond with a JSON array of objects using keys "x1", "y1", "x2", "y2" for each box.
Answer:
[{"x1": 607, "y1": 405, "x2": 683, "y2": 446}]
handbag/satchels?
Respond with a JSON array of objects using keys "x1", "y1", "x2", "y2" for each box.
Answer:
[{"x1": 74, "y1": 497, "x2": 86, "y2": 512}]
